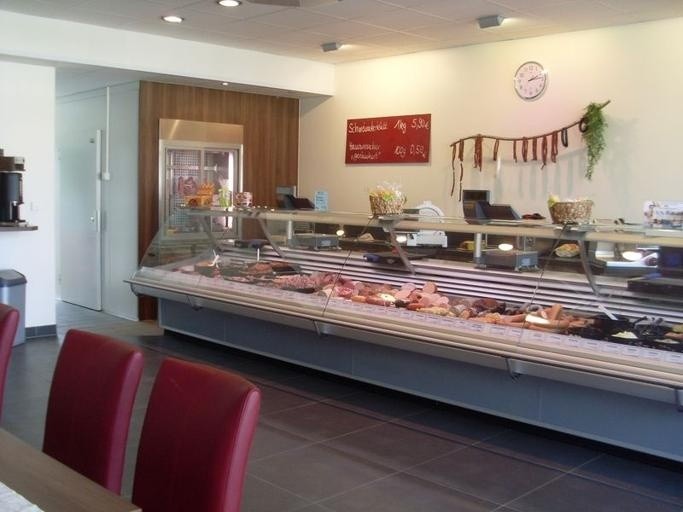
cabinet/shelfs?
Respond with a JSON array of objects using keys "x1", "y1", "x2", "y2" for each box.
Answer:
[{"x1": 122, "y1": 203, "x2": 683, "y2": 472}]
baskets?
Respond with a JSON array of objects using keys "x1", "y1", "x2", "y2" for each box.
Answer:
[{"x1": 546, "y1": 199, "x2": 593, "y2": 225}]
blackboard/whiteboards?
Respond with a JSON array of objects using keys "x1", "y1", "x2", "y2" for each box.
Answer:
[{"x1": 345, "y1": 113, "x2": 429, "y2": 162}]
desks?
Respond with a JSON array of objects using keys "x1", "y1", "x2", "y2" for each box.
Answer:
[{"x1": 0, "y1": 428, "x2": 144, "y2": 512}]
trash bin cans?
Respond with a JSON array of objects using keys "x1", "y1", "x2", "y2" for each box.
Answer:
[{"x1": 0, "y1": 269, "x2": 26, "y2": 350}]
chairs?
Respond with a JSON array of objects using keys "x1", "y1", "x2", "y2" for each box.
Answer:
[
  {"x1": 41, "y1": 328, "x2": 145, "y2": 497},
  {"x1": 128, "y1": 354, "x2": 263, "y2": 512},
  {"x1": 0, "y1": 303, "x2": 21, "y2": 408}
]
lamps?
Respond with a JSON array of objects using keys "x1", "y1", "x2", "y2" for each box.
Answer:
[
  {"x1": 477, "y1": 15, "x2": 503, "y2": 29},
  {"x1": 321, "y1": 42, "x2": 341, "y2": 53}
]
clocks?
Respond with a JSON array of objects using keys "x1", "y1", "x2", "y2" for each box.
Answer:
[{"x1": 512, "y1": 61, "x2": 548, "y2": 101}]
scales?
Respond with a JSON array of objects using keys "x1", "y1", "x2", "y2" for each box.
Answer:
[
  {"x1": 276, "y1": 187, "x2": 338, "y2": 251},
  {"x1": 462, "y1": 189, "x2": 539, "y2": 269}
]
